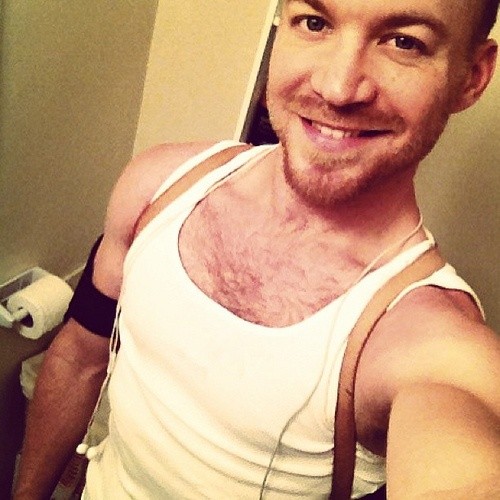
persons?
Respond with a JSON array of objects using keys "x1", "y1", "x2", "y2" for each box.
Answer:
[{"x1": 11, "y1": 0, "x2": 500, "y2": 499}]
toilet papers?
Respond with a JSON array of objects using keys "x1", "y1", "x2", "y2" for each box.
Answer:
[{"x1": 7, "y1": 277, "x2": 75, "y2": 340}]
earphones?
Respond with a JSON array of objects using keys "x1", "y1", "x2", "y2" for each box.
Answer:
[
  {"x1": 76, "y1": 440, "x2": 89, "y2": 455},
  {"x1": 87, "y1": 446, "x2": 98, "y2": 459}
]
